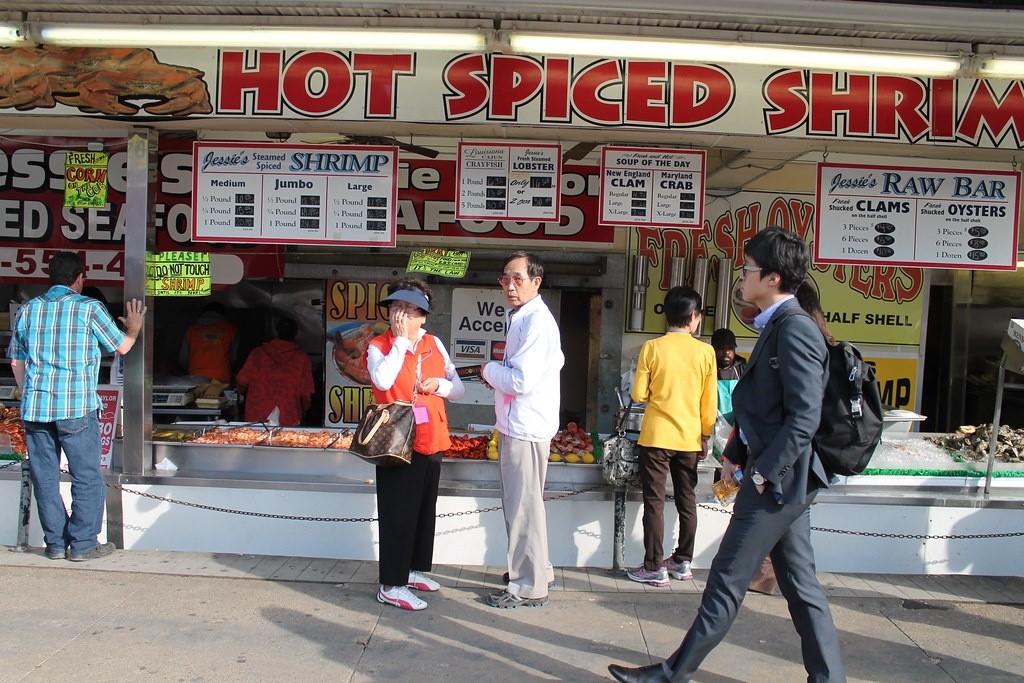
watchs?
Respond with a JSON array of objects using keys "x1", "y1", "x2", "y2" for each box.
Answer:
[{"x1": 751, "y1": 466, "x2": 767, "y2": 486}]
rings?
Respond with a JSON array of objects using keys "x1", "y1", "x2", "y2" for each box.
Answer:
[{"x1": 137, "y1": 311, "x2": 140, "y2": 313}]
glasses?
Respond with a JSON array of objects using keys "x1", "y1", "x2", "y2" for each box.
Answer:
[
  {"x1": 742, "y1": 265, "x2": 763, "y2": 276},
  {"x1": 497, "y1": 276, "x2": 536, "y2": 286}
]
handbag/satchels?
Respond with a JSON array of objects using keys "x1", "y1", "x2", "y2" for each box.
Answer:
[
  {"x1": 348, "y1": 402, "x2": 416, "y2": 465},
  {"x1": 601, "y1": 434, "x2": 641, "y2": 487}
]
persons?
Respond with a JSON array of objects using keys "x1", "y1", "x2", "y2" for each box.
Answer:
[
  {"x1": 720, "y1": 282, "x2": 836, "y2": 596},
  {"x1": 7, "y1": 251, "x2": 147, "y2": 562},
  {"x1": 608, "y1": 225, "x2": 847, "y2": 683},
  {"x1": 179, "y1": 301, "x2": 239, "y2": 396},
  {"x1": 627, "y1": 285, "x2": 718, "y2": 586},
  {"x1": 710, "y1": 329, "x2": 747, "y2": 426},
  {"x1": 235, "y1": 317, "x2": 314, "y2": 427},
  {"x1": 366, "y1": 278, "x2": 465, "y2": 611},
  {"x1": 479, "y1": 251, "x2": 566, "y2": 608}
]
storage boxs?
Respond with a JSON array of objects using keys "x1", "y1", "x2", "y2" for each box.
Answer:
[
  {"x1": 1008, "y1": 318, "x2": 1024, "y2": 352},
  {"x1": 0, "y1": 290, "x2": 31, "y2": 331}
]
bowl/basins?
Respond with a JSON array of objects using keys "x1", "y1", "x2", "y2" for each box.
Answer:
[{"x1": 740, "y1": 306, "x2": 758, "y2": 323}]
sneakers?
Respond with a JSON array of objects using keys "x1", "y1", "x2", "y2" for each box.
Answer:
[
  {"x1": 376, "y1": 583, "x2": 427, "y2": 610},
  {"x1": 503, "y1": 572, "x2": 555, "y2": 589},
  {"x1": 45, "y1": 547, "x2": 68, "y2": 559},
  {"x1": 406, "y1": 570, "x2": 440, "y2": 591},
  {"x1": 627, "y1": 563, "x2": 670, "y2": 586},
  {"x1": 71, "y1": 543, "x2": 116, "y2": 561},
  {"x1": 663, "y1": 556, "x2": 693, "y2": 579},
  {"x1": 487, "y1": 589, "x2": 549, "y2": 609}
]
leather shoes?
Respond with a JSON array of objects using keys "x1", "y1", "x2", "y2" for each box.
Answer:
[{"x1": 608, "y1": 663, "x2": 670, "y2": 683}]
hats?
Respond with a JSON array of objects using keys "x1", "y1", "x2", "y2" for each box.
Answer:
[
  {"x1": 712, "y1": 328, "x2": 737, "y2": 348},
  {"x1": 377, "y1": 287, "x2": 432, "y2": 312}
]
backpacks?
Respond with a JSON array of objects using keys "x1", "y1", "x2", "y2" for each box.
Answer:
[{"x1": 768, "y1": 307, "x2": 884, "y2": 476}]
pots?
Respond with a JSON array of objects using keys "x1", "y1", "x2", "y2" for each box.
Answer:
[{"x1": 613, "y1": 405, "x2": 645, "y2": 432}]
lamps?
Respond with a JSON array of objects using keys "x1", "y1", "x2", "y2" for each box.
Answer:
[
  {"x1": 0, "y1": 11, "x2": 499, "y2": 55},
  {"x1": 499, "y1": 20, "x2": 1024, "y2": 82}
]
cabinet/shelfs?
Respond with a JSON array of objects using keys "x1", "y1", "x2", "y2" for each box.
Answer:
[{"x1": 0, "y1": 330, "x2": 17, "y2": 386}]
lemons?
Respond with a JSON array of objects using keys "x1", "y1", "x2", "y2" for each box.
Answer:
[{"x1": 488, "y1": 428, "x2": 594, "y2": 463}]
